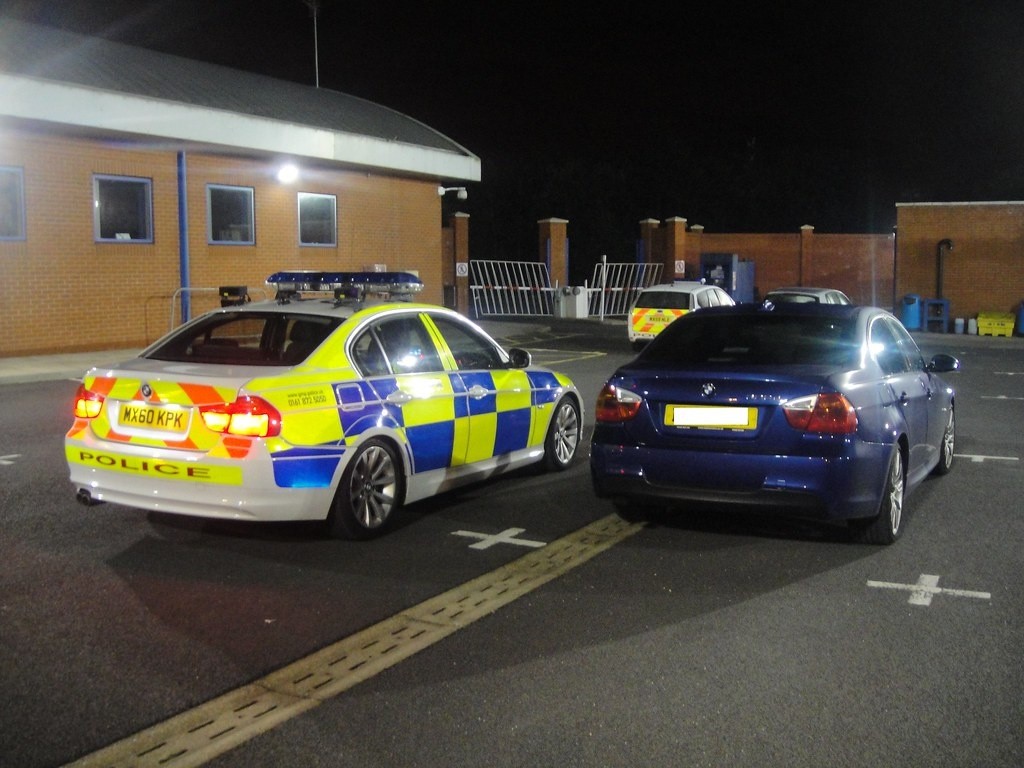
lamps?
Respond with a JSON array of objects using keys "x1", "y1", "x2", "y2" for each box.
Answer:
[{"x1": 438, "y1": 186, "x2": 467, "y2": 199}]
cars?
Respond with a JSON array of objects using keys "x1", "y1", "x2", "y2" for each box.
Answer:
[
  {"x1": 626, "y1": 278, "x2": 737, "y2": 349},
  {"x1": 760, "y1": 285, "x2": 851, "y2": 309},
  {"x1": 588, "y1": 300, "x2": 961, "y2": 546},
  {"x1": 66, "y1": 258, "x2": 583, "y2": 541}
]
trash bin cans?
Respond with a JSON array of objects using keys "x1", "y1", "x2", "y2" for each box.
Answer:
[{"x1": 899, "y1": 293, "x2": 921, "y2": 331}]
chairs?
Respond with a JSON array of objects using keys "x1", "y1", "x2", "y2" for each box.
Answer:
[{"x1": 193, "y1": 321, "x2": 338, "y2": 366}]
